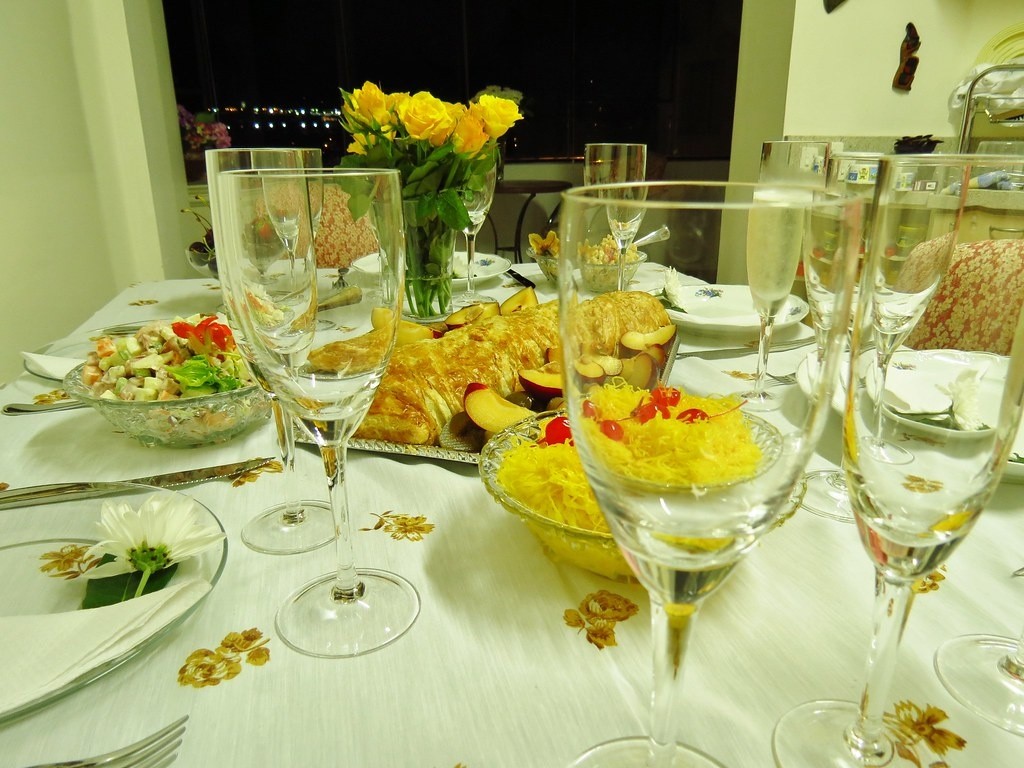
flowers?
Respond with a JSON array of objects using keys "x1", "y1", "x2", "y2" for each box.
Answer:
[{"x1": 337, "y1": 80, "x2": 522, "y2": 198}]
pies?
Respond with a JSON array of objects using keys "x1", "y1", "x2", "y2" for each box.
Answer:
[{"x1": 336, "y1": 291, "x2": 672, "y2": 445}]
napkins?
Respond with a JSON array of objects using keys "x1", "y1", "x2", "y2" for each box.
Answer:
[{"x1": 18, "y1": 350, "x2": 86, "y2": 379}]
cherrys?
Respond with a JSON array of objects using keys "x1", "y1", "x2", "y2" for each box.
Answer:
[{"x1": 541, "y1": 386, "x2": 749, "y2": 446}]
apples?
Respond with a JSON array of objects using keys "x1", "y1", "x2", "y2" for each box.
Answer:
[
  {"x1": 516, "y1": 368, "x2": 564, "y2": 398},
  {"x1": 371, "y1": 308, "x2": 443, "y2": 345},
  {"x1": 445, "y1": 301, "x2": 501, "y2": 329},
  {"x1": 588, "y1": 356, "x2": 622, "y2": 374},
  {"x1": 620, "y1": 323, "x2": 678, "y2": 350},
  {"x1": 501, "y1": 285, "x2": 538, "y2": 317},
  {"x1": 575, "y1": 360, "x2": 608, "y2": 385},
  {"x1": 626, "y1": 353, "x2": 661, "y2": 391},
  {"x1": 462, "y1": 383, "x2": 538, "y2": 433},
  {"x1": 620, "y1": 344, "x2": 665, "y2": 378}
]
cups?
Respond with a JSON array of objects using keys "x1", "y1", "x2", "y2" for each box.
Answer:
[{"x1": 583, "y1": 143, "x2": 648, "y2": 186}]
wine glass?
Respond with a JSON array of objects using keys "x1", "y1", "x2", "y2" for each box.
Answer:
[
  {"x1": 451, "y1": 156, "x2": 498, "y2": 306},
  {"x1": 185, "y1": 244, "x2": 230, "y2": 314},
  {"x1": 205, "y1": 148, "x2": 337, "y2": 555},
  {"x1": 771, "y1": 154, "x2": 1024, "y2": 768},
  {"x1": 217, "y1": 168, "x2": 421, "y2": 658},
  {"x1": 250, "y1": 149, "x2": 306, "y2": 292},
  {"x1": 934, "y1": 626, "x2": 1024, "y2": 737},
  {"x1": 241, "y1": 241, "x2": 285, "y2": 286},
  {"x1": 558, "y1": 181, "x2": 864, "y2": 768},
  {"x1": 722, "y1": 142, "x2": 970, "y2": 524},
  {"x1": 599, "y1": 189, "x2": 647, "y2": 292}
]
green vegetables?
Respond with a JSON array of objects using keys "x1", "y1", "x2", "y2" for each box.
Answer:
[{"x1": 154, "y1": 353, "x2": 245, "y2": 400}]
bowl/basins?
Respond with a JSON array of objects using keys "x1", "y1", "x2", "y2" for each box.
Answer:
[
  {"x1": 478, "y1": 410, "x2": 806, "y2": 587},
  {"x1": 525, "y1": 246, "x2": 646, "y2": 292}
]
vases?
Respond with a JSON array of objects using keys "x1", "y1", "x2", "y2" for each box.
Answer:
[{"x1": 374, "y1": 199, "x2": 459, "y2": 321}]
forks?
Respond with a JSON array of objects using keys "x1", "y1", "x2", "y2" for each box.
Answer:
[
  {"x1": 765, "y1": 341, "x2": 876, "y2": 386},
  {"x1": 25, "y1": 714, "x2": 189, "y2": 768}
]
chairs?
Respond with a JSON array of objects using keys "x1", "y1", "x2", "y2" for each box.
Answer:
[
  {"x1": 897, "y1": 234, "x2": 1024, "y2": 352},
  {"x1": 254, "y1": 183, "x2": 381, "y2": 269}
]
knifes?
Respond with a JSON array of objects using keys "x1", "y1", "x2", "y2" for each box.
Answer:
[
  {"x1": 674, "y1": 336, "x2": 815, "y2": 360},
  {"x1": 0, "y1": 457, "x2": 275, "y2": 510},
  {"x1": 506, "y1": 269, "x2": 536, "y2": 289}
]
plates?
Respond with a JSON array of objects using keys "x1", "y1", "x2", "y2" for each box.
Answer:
[
  {"x1": 63, "y1": 361, "x2": 272, "y2": 449},
  {"x1": 352, "y1": 250, "x2": 511, "y2": 291},
  {"x1": 795, "y1": 348, "x2": 1024, "y2": 484},
  {"x1": 23, "y1": 319, "x2": 173, "y2": 382},
  {"x1": 642, "y1": 284, "x2": 808, "y2": 338},
  {"x1": 0, "y1": 482, "x2": 228, "y2": 723}
]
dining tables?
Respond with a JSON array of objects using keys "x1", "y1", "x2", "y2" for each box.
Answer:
[{"x1": 0, "y1": 278, "x2": 1024, "y2": 768}]
